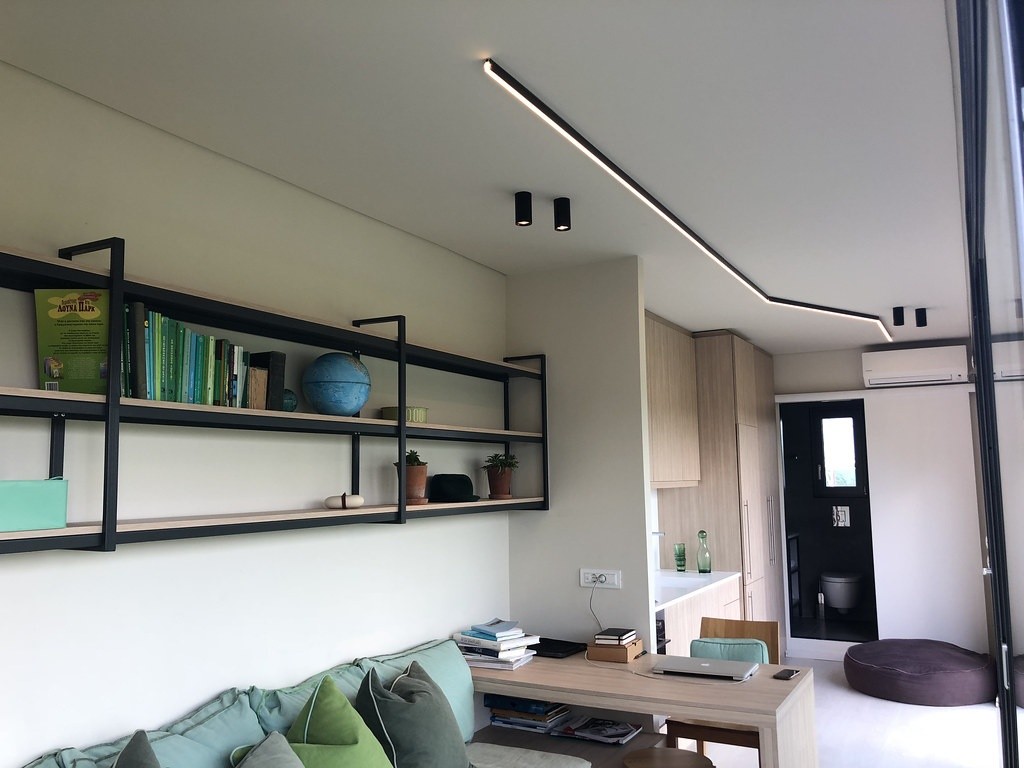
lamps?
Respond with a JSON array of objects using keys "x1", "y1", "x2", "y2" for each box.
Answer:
[
  {"x1": 514, "y1": 189, "x2": 533, "y2": 226},
  {"x1": 916, "y1": 307, "x2": 928, "y2": 327},
  {"x1": 553, "y1": 197, "x2": 571, "y2": 232},
  {"x1": 892, "y1": 307, "x2": 906, "y2": 326}
]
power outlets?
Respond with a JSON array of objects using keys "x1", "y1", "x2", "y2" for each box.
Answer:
[{"x1": 580, "y1": 569, "x2": 622, "y2": 590}]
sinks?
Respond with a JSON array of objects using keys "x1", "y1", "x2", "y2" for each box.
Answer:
[{"x1": 656, "y1": 568, "x2": 741, "y2": 612}]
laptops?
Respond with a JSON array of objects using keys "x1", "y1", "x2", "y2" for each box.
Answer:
[{"x1": 653, "y1": 655, "x2": 758, "y2": 681}]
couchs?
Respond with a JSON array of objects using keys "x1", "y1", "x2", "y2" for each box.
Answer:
[{"x1": 24, "y1": 637, "x2": 596, "y2": 768}]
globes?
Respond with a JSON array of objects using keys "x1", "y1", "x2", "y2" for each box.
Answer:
[
  {"x1": 283, "y1": 389, "x2": 297, "y2": 411},
  {"x1": 302, "y1": 352, "x2": 371, "y2": 415}
]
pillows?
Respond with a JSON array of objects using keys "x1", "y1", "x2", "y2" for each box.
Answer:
[
  {"x1": 691, "y1": 638, "x2": 770, "y2": 663},
  {"x1": 115, "y1": 661, "x2": 494, "y2": 768}
]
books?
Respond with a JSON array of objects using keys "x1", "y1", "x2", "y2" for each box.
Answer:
[
  {"x1": 452, "y1": 618, "x2": 540, "y2": 671},
  {"x1": 593, "y1": 627, "x2": 637, "y2": 645},
  {"x1": 34, "y1": 288, "x2": 268, "y2": 410},
  {"x1": 490, "y1": 704, "x2": 643, "y2": 744}
]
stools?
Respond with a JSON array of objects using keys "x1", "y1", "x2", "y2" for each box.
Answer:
[{"x1": 845, "y1": 640, "x2": 995, "y2": 707}]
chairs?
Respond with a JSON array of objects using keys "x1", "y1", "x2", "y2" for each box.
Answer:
[{"x1": 664, "y1": 617, "x2": 778, "y2": 768}]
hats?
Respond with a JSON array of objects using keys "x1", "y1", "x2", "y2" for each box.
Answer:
[{"x1": 428, "y1": 474, "x2": 480, "y2": 503}]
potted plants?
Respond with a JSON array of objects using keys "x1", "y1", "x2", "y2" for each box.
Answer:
[
  {"x1": 482, "y1": 450, "x2": 519, "y2": 500},
  {"x1": 392, "y1": 449, "x2": 429, "y2": 503}
]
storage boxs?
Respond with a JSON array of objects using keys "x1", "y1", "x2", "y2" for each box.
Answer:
[{"x1": 0, "y1": 477, "x2": 70, "y2": 532}]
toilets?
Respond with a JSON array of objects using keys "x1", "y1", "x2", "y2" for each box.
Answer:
[{"x1": 819, "y1": 571, "x2": 867, "y2": 621}]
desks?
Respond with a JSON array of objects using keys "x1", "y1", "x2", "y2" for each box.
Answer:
[{"x1": 467, "y1": 640, "x2": 815, "y2": 768}]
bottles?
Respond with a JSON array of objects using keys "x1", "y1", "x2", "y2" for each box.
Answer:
[{"x1": 697, "y1": 531, "x2": 711, "y2": 573}]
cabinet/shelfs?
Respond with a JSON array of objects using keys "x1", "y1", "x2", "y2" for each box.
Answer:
[
  {"x1": 664, "y1": 579, "x2": 740, "y2": 656},
  {"x1": 0, "y1": 238, "x2": 548, "y2": 552},
  {"x1": 733, "y1": 328, "x2": 788, "y2": 660},
  {"x1": 786, "y1": 533, "x2": 803, "y2": 623}
]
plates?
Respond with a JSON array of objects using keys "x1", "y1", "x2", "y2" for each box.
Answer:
[{"x1": 428, "y1": 496, "x2": 479, "y2": 502}]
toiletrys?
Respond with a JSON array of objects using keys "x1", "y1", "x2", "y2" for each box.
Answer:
[
  {"x1": 696, "y1": 530, "x2": 712, "y2": 574},
  {"x1": 673, "y1": 542, "x2": 686, "y2": 572}
]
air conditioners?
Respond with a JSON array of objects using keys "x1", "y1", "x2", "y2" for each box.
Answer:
[
  {"x1": 991, "y1": 340, "x2": 1024, "y2": 381},
  {"x1": 862, "y1": 345, "x2": 968, "y2": 387}
]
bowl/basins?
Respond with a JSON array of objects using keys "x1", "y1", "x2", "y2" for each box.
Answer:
[{"x1": 432, "y1": 474, "x2": 473, "y2": 496}]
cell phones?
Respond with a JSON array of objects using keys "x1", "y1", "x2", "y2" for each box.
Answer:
[{"x1": 773, "y1": 669, "x2": 800, "y2": 680}]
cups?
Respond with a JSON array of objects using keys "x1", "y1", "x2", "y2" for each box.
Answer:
[{"x1": 673, "y1": 543, "x2": 686, "y2": 571}]
